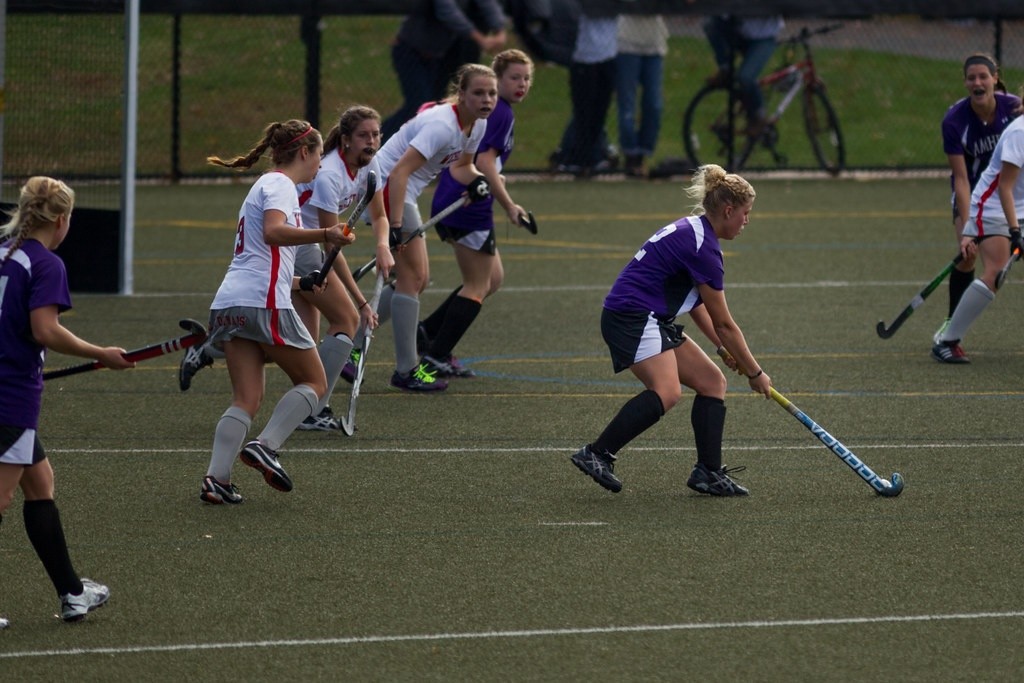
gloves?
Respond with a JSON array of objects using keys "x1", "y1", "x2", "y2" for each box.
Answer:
[{"x1": 1008, "y1": 227, "x2": 1024, "y2": 261}]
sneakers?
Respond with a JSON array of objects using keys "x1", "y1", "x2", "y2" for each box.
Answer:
[
  {"x1": 931, "y1": 339, "x2": 970, "y2": 363},
  {"x1": 297, "y1": 407, "x2": 359, "y2": 432},
  {"x1": 420, "y1": 356, "x2": 476, "y2": 377},
  {"x1": 59, "y1": 578, "x2": 109, "y2": 619},
  {"x1": 240, "y1": 439, "x2": 292, "y2": 492},
  {"x1": 340, "y1": 348, "x2": 365, "y2": 385},
  {"x1": 180, "y1": 335, "x2": 214, "y2": 390},
  {"x1": 570, "y1": 444, "x2": 622, "y2": 493},
  {"x1": 390, "y1": 362, "x2": 449, "y2": 393},
  {"x1": 687, "y1": 463, "x2": 749, "y2": 496},
  {"x1": 200, "y1": 476, "x2": 242, "y2": 504}
]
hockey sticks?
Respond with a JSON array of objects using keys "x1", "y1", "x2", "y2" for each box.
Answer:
[
  {"x1": 517, "y1": 211, "x2": 541, "y2": 237},
  {"x1": 715, "y1": 344, "x2": 905, "y2": 499},
  {"x1": 43, "y1": 319, "x2": 209, "y2": 382},
  {"x1": 339, "y1": 269, "x2": 384, "y2": 436},
  {"x1": 877, "y1": 237, "x2": 978, "y2": 340},
  {"x1": 315, "y1": 171, "x2": 376, "y2": 287},
  {"x1": 352, "y1": 190, "x2": 476, "y2": 280},
  {"x1": 995, "y1": 248, "x2": 1019, "y2": 292}
]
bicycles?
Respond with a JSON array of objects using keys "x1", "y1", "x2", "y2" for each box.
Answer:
[{"x1": 683, "y1": 23, "x2": 850, "y2": 170}]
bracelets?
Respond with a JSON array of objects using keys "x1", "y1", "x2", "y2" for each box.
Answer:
[
  {"x1": 358, "y1": 300, "x2": 369, "y2": 310},
  {"x1": 747, "y1": 370, "x2": 764, "y2": 380},
  {"x1": 324, "y1": 227, "x2": 329, "y2": 243},
  {"x1": 391, "y1": 220, "x2": 401, "y2": 226}
]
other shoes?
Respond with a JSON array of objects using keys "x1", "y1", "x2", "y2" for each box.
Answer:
[{"x1": 933, "y1": 321, "x2": 961, "y2": 352}]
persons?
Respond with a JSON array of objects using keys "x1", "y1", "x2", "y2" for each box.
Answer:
[
  {"x1": 700, "y1": 0, "x2": 790, "y2": 151},
  {"x1": 178, "y1": 104, "x2": 396, "y2": 432},
  {"x1": 378, "y1": 0, "x2": 516, "y2": 145},
  {"x1": 537, "y1": 0, "x2": 670, "y2": 179},
  {"x1": 569, "y1": 163, "x2": 774, "y2": 497},
  {"x1": 418, "y1": 48, "x2": 534, "y2": 378},
  {"x1": 0, "y1": 175, "x2": 137, "y2": 631},
  {"x1": 340, "y1": 65, "x2": 499, "y2": 393},
  {"x1": 196, "y1": 120, "x2": 357, "y2": 507},
  {"x1": 930, "y1": 55, "x2": 1024, "y2": 366}
]
